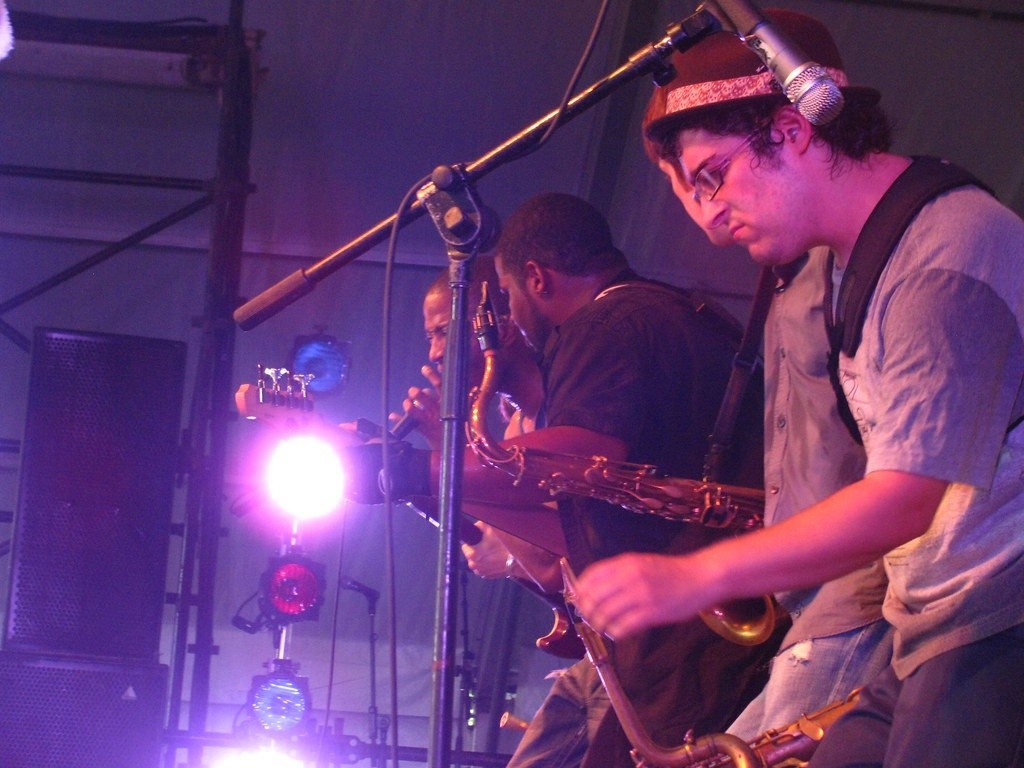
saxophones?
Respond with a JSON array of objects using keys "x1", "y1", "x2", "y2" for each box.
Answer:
[
  {"x1": 469, "y1": 280, "x2": 791, "y2": 648},
  {"x1": 556, "y1": 556, "x2": 864, "y2": 768}
]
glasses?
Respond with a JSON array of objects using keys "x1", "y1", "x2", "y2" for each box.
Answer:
[{"x1": 692, "y1": 114, "x2": 774, "y2": 207}]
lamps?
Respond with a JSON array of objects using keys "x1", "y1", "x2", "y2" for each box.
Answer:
[{"x1": 229, "y1": 328, "x2": 352, "y2": 755}]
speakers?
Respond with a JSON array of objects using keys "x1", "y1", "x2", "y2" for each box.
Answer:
[{"x1": 1, "y1": 325, "x2": 184, "y2": 768}]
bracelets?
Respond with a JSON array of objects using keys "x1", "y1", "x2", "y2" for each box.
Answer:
[{"x1": 505, "y1": 553, "x2": 515, "y2": 578}]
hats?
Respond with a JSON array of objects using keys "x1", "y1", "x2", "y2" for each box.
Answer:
[{"x1": 641, "y1": 8, "x2": 881, "y2": 146}]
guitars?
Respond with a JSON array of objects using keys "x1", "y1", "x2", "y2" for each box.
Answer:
[
  {"x1": 233, "y1": 363, "x2": 571, "y2": 555},
  {"x1": 341, "y1": 415, "x2": 586, "y2": 659}
]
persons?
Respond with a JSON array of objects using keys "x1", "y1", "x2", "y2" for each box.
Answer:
[
  {"x1": 564, "y1": 9, "x2": 1024, "y2": 767},
  {"x1": 336, "y1": 192, "x2": 794, "y2": 768}
]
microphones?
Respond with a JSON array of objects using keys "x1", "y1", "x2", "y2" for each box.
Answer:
[
  {"x1": 718, "y1": 0, "x2": 845, "y2": 126},
  {"x1": 341, "y1": 576, "x2": 381, "y2": 601},
  {"x1": 389, "y1": 388, "x2": 440, "y2": 441}
]
